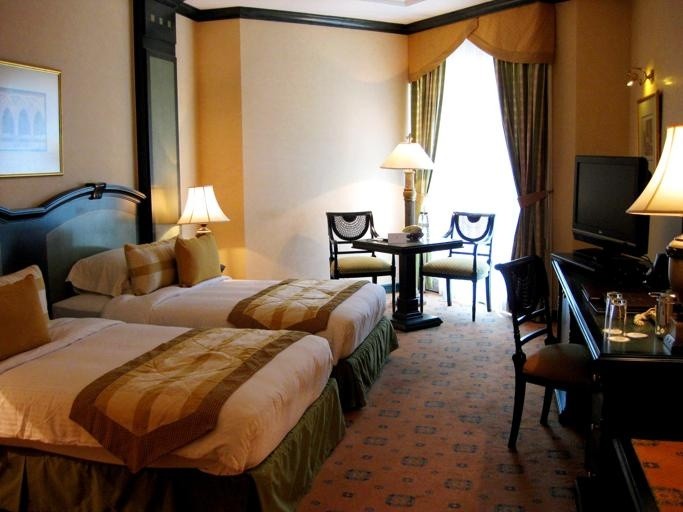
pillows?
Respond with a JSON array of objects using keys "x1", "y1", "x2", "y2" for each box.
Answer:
[
  {"x1": 125, "y1": 233, "x2": 187, "y2": 296},
  {"x1": 172, "y1": 230, "x2": 223, "y2": 291},
  {"x1": 0, "y1": 271, "x2": 51, "y2": 363},
  {"x1": 0, "y1": 263, "x2": 51, "y2": 327},
  {"x1": 63, "y1": 246, "x2": 132, "y2": 299}
]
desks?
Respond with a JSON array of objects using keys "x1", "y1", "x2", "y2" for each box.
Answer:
[
  {"x1": 612, "y1": 430, "x2": 682, "y2": 512},
  {"x1": 351, "y1": 230, "x2": 464, "y2": 335},
  {"x1": 550, "y1": 245, "x2": 682, "y2": 477}
]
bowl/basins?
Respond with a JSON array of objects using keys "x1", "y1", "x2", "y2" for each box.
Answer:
[{"x1": 406, "y1": 233, "x2": 423, "y2": 240}]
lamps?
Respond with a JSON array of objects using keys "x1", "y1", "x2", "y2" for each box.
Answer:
[
  {"x1": 174, "y1": 184, "x2": 232, "y2": 238},
  {"x1": 624, "y1": 64, "x2": 656, "y2": 91},
  {"x1": 625, "y1": 124, "x2": 683, "y2": 316},
  {"x1": 378, "y1": 131, "x2": 437, "y2": 228}
]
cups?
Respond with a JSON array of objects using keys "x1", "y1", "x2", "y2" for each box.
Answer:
[
  {"x1": 603, "y1": 291, "x2": 621, "y2": 330},
  {"x1": 609, "y1": 299, "x2": 627, "y2": 334}
]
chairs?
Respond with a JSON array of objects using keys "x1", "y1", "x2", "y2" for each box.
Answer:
[
  {"x1": 492, "y1": 253, "x2": 600, "y2": 476},
  {"x1": 323, "y1": 211, "x2": 396, "y2": 319},
  {"x1": 418, "y1": 210, "x2": 496, "y2": 323}
]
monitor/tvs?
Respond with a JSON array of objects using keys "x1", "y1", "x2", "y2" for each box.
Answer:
[{"x1": 572, "y1": 155, "x2": 652, "y2": 268}]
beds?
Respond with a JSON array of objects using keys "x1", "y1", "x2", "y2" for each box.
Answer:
[
  {"x1": 45, "y1": 180, "x2": 400, "y2": 414},
  {"x1": 0, "y1": 208, "x2": 354, "y2": 511}
]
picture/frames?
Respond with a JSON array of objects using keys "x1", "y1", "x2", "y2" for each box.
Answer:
[
  {"x1": 0, "y1": 60, "x2": 64, "y2": 179},
  {"x1": 633, "y1": 90, "x2": 661, "y2": 175}
]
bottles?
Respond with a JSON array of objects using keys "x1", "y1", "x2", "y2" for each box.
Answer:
[{"x1": 655, "y1": 293, "x2": 679, "y2": 338}]
